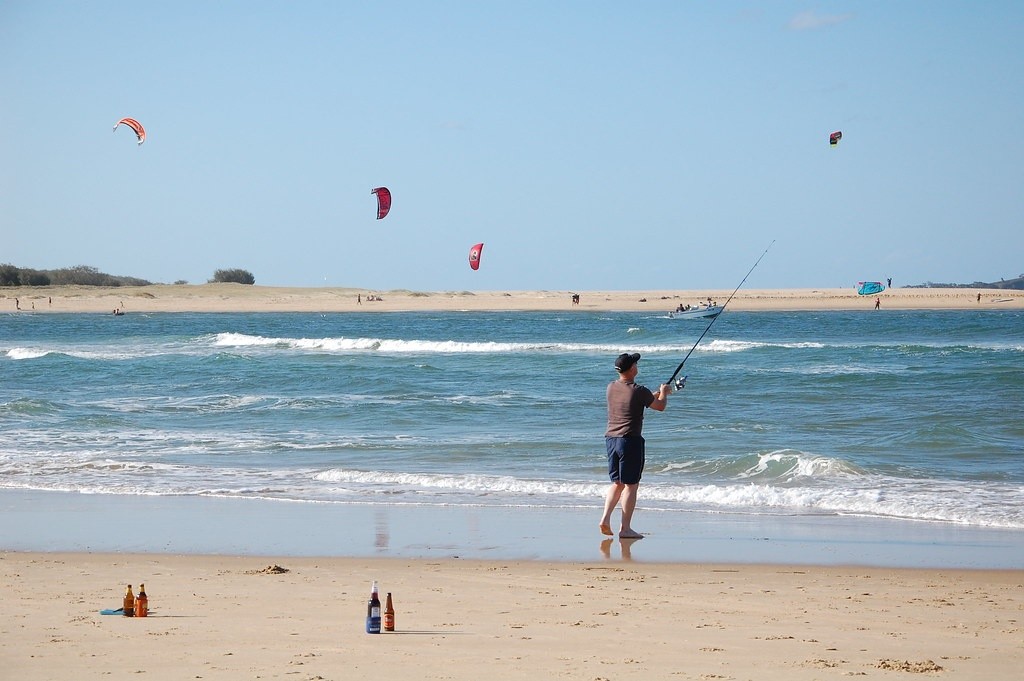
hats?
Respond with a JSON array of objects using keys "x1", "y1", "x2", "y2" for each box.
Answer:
[{"x1": 615, "y1": 353, "x2": 640, "y2": 374}]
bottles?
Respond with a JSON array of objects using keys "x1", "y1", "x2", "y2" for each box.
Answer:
[
  {"x1": 135, "y1": 583, "x2": 147, "y2": 617},
  {"x1": 124, "y1": 584, "x2": 135, "y2": 618},
  {"x1": 384, "y1": 592, "x2": 395, "y2": 631},
  {"x1": 366, "y1": 581, "x2": 381, "y2": 634}
]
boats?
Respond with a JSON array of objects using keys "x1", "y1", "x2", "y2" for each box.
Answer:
[{"x1": 668, "y1": 306, "x2": 723, "y2": 319}]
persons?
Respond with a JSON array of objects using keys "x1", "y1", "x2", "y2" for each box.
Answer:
[
  {"x1": 572, "y1": 294, "x2": 579, "y2": 304},
  {"x1": 357, "y1": 294, "x2": 361, "y2": 304},
  {"x1": 676, "y1": 304, "x2": 691, "y2": 311},
  {"x1": 600, "y1": 353, "x2": 671, "y2": 538},
  {"x1": 708, "y1": 301, "x2": 716, "y2": 308},
  {"x1": 977, "y1": 293, "x2": 981, "y2": 301},
  {"x1": 16, "y1": 298, "x2": 19, "y2": 308},
  {"x1": 875, "y1": 298, "x2": 880, "y2": 310},
  {"x1": 887, "y1": 278, "x2": 892, "y2": 288}
]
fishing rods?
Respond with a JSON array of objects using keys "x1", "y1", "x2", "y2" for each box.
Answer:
[{"x1": 658, "y1": 229, "x2": 784, "y2": 396}]
